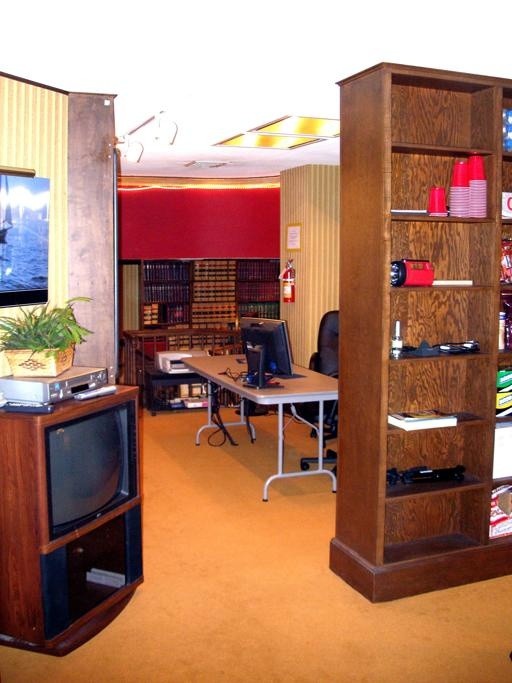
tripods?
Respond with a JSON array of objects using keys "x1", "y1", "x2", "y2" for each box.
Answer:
[{"x1": 389, "y1": 463, "x2": 466, "y2": 484}]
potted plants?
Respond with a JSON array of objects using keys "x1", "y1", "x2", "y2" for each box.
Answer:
[{"x1": 0, "y1": 295, "x2": 97, "y2": 378}]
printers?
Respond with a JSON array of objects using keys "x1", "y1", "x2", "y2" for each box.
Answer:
[{"x1": 157, "y1": 349, "x2": 211, "y2": 374}]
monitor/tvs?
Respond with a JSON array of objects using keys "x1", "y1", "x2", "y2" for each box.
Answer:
[
  {"x1": 45, "y1": 398, "x2": 138, "y2": 541},
  {"x1": 239, "y1": 316, "x2": 293, "y2": 392}
]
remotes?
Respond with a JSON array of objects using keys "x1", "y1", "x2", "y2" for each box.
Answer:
[
  {"x1": 4, "y1": 401, "x2": 53, "y2": 414},
  {"x1": 74, "y1": 384, "x2": 116, "y2": 401}
]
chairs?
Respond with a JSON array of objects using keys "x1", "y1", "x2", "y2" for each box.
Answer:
[{"x1": 298, "y1": 309, "x2": 339, "y2": 475}]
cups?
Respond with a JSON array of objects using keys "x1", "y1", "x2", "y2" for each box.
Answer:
[{"x1": 428, "y1": 156, "x2": 489, "y2": 217}]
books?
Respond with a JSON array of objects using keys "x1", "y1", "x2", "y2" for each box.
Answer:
[
  {"x1": 391, "y1": 208, "x2": 429, "y2": 216},
  {"x1": 432, "y1": 278, "x2": 474, "y2": 286},
  {"x1": 388, "y1": 409, "x2": 458, "y2": 432},
  {"x1": 143, "y1": 259, "x2": 280, "y2": 408}
]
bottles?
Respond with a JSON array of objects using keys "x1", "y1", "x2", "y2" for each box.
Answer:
[{"x1": 390, "y1": 319, "x2": 405, "y2": 359}]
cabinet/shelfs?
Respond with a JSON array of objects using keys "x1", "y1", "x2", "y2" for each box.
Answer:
[
  {"x1": 3, "y1": 383, "x2": 147, "y2": 659},
  {"x1": 326, "y1": 60, "x2": 512, "y2": 605},
  {"x1": 123, "y1": 258, "x2": 281, "y2": 416}
]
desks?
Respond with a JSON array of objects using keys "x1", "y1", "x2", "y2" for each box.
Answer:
[{"x1": 180, "y1": 354, "x2": 339, "y2": 502}]
